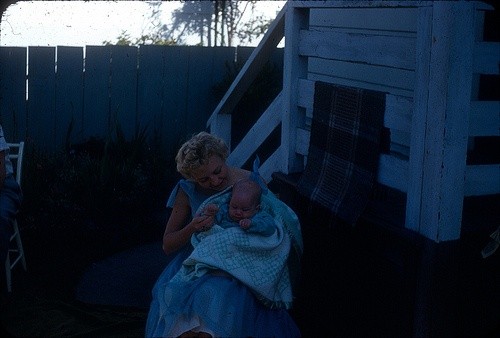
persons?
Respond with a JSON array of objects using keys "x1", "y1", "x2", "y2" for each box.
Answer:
[
  {"x1": 200, "y1": 179, "x2": 277, "y2": 277},
  {"x1": 144, "y1": 131, "x2": 303, "y2": 338},
  {"x1": 0, "y1": 123, "x2": 24, "y2": 322}
]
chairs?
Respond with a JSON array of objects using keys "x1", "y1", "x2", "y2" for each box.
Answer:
[{"x1": 6, "y1": 141, "x2": 27, "y2": 294}]
